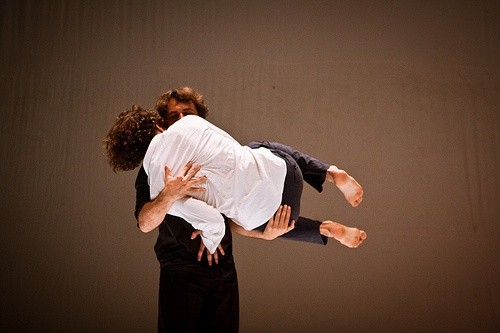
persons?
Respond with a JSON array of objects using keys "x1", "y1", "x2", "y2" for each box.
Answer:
[
  {"x1": 134, "y1": 87, "x2": 239, "y2": 333},
  {"x1": 102, "y1": 106, "x2": 367, "y2": 266}
]
